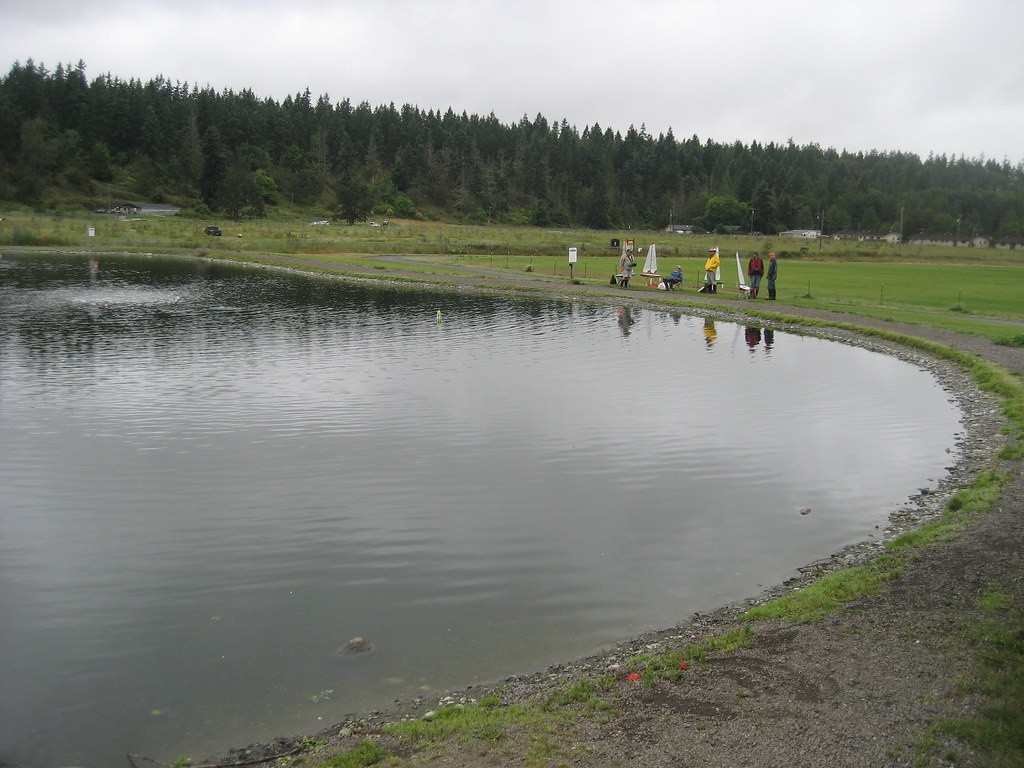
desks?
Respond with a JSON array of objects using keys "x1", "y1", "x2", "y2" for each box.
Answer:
[{"x1": 640, "y1": 273, "x2": 661, "y2": 287}]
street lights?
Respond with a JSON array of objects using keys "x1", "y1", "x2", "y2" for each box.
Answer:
[
  {"x1": 816, "y1": 216, "x2": 819, "y2": 238},
  {"x1": 751, "y1": 208, "x2": 755, "y2": 235}
]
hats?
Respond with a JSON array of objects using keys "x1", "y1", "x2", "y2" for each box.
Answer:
[
  {"x1": 754, "y1": 252, "x2": 759, "y2": 255},
  {"x1": 627, "y1": 248, "x2": 632, "y2": 251},
  {"x1": 676, "y1": 265, "x2": 681, "y2": 269},
  {"x1": 708, "y1": 249, "x2": 716, "y2": 252},
  {"x1": 768, "y1": 253, "x2": 775, "y2": 256}
]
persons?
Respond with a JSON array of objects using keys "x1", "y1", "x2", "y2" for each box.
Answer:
[
  {"x1": 620, "y1": 248, "x2": 635, "y2": 287},
  {"x1": 662, "y1": 265, "x2": 683, "y2": 291},
  {"x1": 705, "y1": 249, "x2": 720, "y2": 294},
  {"x1": 748, "y1": 251, "x2": 764, "y2": 297},
  {"x1": 765, "y1": 253, "x2": 778, "y2": 300}
]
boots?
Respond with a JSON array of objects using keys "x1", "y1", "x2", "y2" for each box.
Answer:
[
  {"x1": 621, "y1": 279, "x2": 624, "y2": 286},
  {"x1": 706, "y1": 284, "x2": 712, "y2": 292},
  {"x1": 711, "y1": 285, "x2": 716, "y2": 294},
  {"x1": 625, "y1": 280, "x2": 628, "y2": 287},
  {"x1": 765, "y1": 289, "x2": 776, "y2": 300}
]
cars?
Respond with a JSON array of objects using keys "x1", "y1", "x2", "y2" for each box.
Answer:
[{"x1": 205, "y1": 226, "x2": 221, "y2": 236}]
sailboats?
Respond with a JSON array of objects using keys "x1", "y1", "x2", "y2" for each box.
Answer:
[
  {"x1": 702, "y1": 247, "x2": 724, "y2": 284},
  {"x1": 640, "y1": 244, "x2": 661, "y2": 278},
  {"x1": 736, "y1": 250, "x2": 751, "y2": 291}
]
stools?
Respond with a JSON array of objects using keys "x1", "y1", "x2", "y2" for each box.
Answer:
[
  {"x1": 701, "y1": 280, "x2": 724, "y2": 293},
  {"x1": 737, "y1": 286, "x2": 757, "y2": 300},
  {"x1": 616, "y1": 272, "x2": 635, "y2": 287}
]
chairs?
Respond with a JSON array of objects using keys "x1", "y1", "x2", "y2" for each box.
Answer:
[{"x1": 669, "y1": 278, "x2": 682, "y2": 291}]
